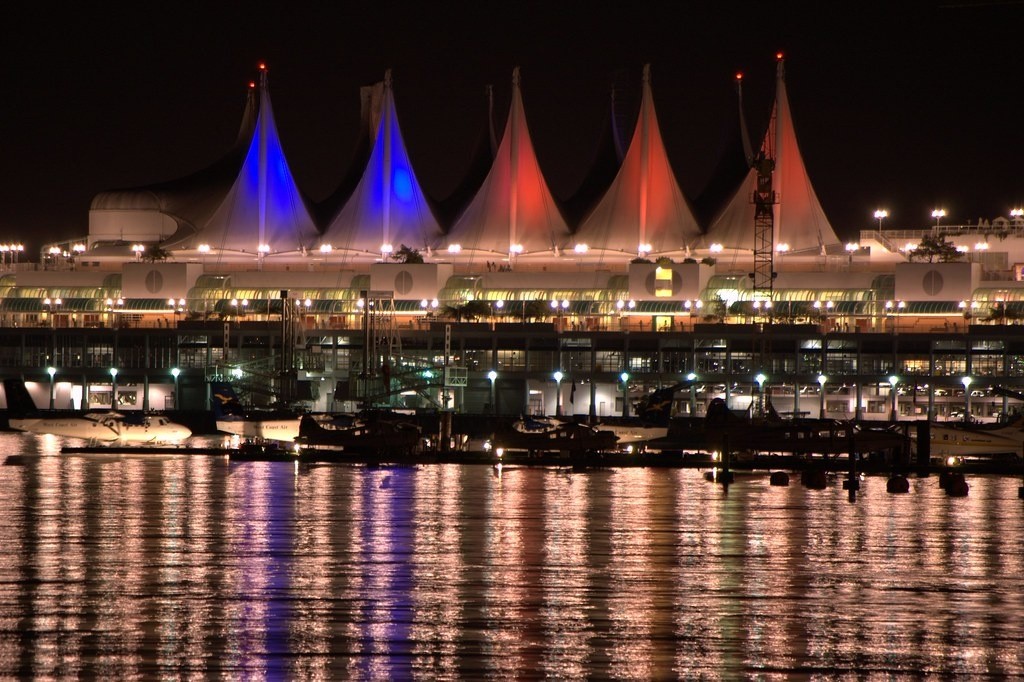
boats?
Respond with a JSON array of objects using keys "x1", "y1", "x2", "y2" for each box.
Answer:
[{"x1": 0, "y1": 286, "x2": 1023, "y2": 501}]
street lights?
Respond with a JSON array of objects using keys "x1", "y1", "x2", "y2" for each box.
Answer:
[
  {"x1": 931, "y1": 208, "x2": 946, "y2": 228},
  {"x1": 132, "y1": 244, "x2": 144, "y2": 262},
  {"x1": 0, "y1": 243, "x2": 24, "y2": 271},
  {"x1": 875, "y1": 210, "x2": 887, "y2": 235}
]
